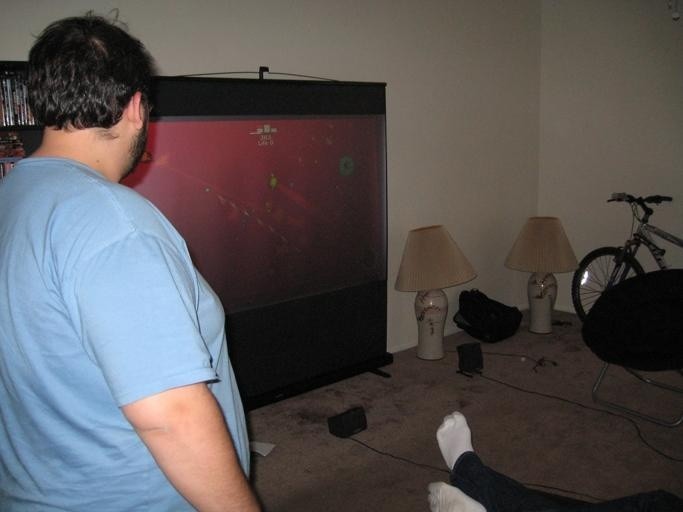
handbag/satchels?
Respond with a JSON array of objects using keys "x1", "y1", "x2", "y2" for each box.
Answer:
[{"x1": 452, "y1": 288, "x2": 523, "y2": 343}]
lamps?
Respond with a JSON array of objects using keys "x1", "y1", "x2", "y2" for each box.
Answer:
[
  {"x1": 503, "y1": 216, "x2": 582, "y2": 334},
  {"x1": 393, "y1": 224, "x2": 477, "y2": 361}
]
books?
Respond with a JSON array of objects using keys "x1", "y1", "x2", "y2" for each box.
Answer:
[{"x1": 0, "y1": 67, "x2": 40, "y2": 181}]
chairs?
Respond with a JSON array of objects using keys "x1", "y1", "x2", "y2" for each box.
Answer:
[{"x1": 582, "y1": 269, "x2": 683, "y2": 427}]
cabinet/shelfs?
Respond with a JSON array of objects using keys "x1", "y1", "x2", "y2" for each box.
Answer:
[{"x1": 0, "y1": 60, "x2": 43, "y2": 179}]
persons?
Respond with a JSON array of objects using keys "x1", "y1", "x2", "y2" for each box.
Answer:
[
  {"x1": 426, "y1": 410, "x2": 681, "y2": 511},
  {"x1": 0, "y1": 7, "x2": 264, "y2": 512}
]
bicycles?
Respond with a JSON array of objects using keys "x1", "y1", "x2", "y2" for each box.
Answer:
[{"x1": 568, "y1": 187, "x2": 682, "y2": 329}]
